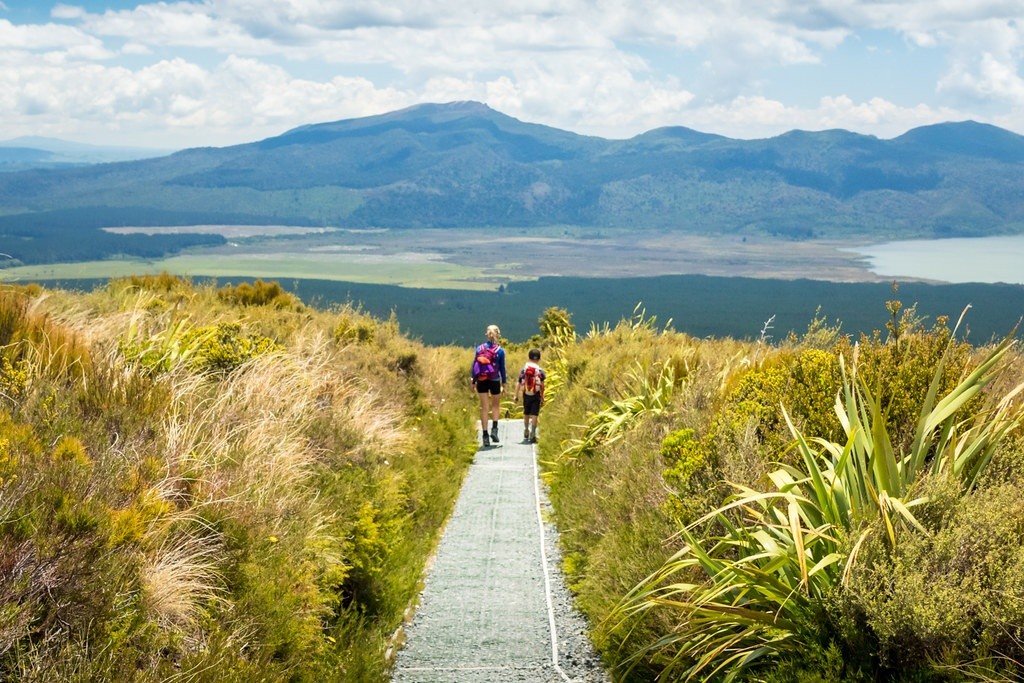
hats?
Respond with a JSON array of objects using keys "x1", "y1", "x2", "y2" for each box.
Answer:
[{"x1": 529, "y1": 349, "x2": 540, "y2": 360}]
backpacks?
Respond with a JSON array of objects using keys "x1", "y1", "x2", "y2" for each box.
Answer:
[
  {"x1": 473, "y1": 343, "x2": 501, "y2": 382},
  {"x1": 520, "y1": 362, "x2": 545, "y2": 402}
]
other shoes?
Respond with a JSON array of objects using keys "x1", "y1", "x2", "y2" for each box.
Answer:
[
  {"x1": 483, "y1": 436, "x2": 490, "y2": 446},
  {"x1": 530, "y1": 437, "x2": 536, "y2": 443},
  {"x1": 490, "y1": 430, "x2": 499, "y2": 442},
  {"x1": 524, "y1": 430, "x2": 528, "y2": 438}
]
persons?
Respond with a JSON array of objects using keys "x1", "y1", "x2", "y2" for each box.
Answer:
[
  {"x1": 471, "y1": 325, "x2": 507, "y2": 446},
  {"x1": 514, "y1": 349, "x2": 546, "y2": 443}
]
